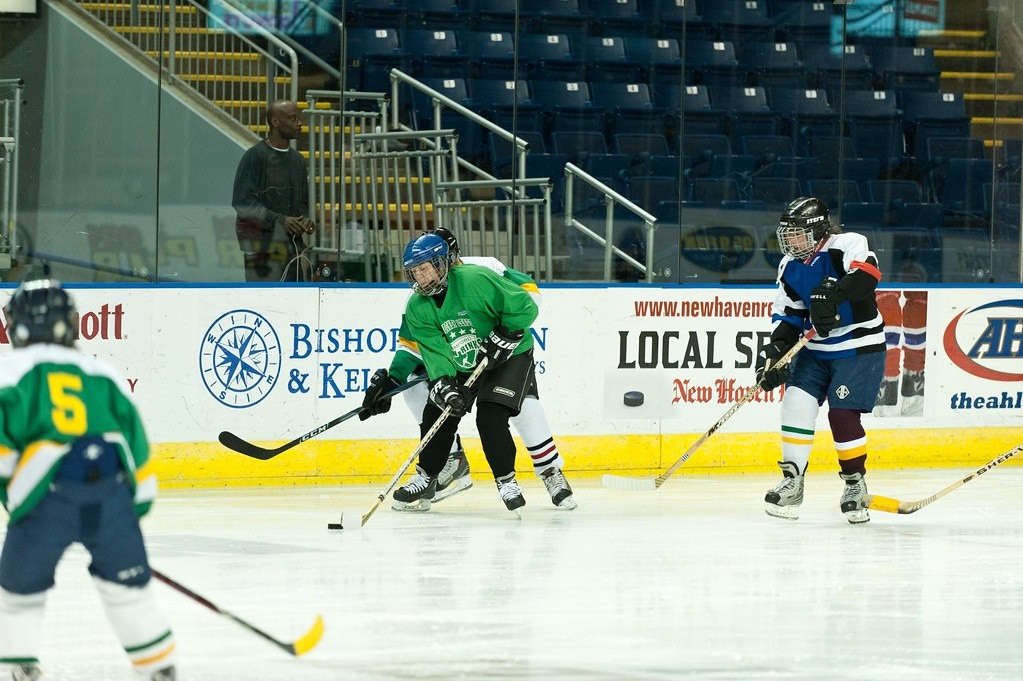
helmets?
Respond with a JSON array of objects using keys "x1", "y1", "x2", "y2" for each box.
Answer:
[
  {"x1": 6, "y1": 277, "x2": 79, "y2": 348},
  {"x1": 776, "y1": 197, "x2": 831, "y2": 261},
  {"x1": 421, "y1": 226, "x2": 461, "y2": 267},
  {"x1": 402, "y1": 234, "x2": 449, "y2": 297}
]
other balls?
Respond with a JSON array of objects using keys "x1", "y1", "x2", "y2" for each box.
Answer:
[
  {"x1": 623, "y1": 391, "x2": 644, "y2": 407},
  {"x1": 328, "y1": 524, "x2": 343, "y2": 530}
]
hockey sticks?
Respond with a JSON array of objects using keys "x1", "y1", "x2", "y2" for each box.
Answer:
[
  {"x1": 340, "y1": 355, "x2": 491, "y2": 528},
  {"x1": 218, "y1": 372, "x2": 430, "y2": 460},
  {"x1": 857, "y1": 443, "x2": 1023, "y2": 514},
  {"x1": 149, "y1": 567, "x2": 325, "y2": 657},
  {"x1": 602, "y1": 327, "x2": 817, "y2": 492}
]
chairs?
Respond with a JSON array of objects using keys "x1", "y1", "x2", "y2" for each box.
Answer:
[{"x1": 335, "y1": 1, "x2": 1022, "y2": 287}]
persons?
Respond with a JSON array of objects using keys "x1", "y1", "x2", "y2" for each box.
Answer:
[
  {"x1": 756, "y1": 197, "x2": 887, "y2": 524},
  {"x1": 357, "y1": 228, "x2": 579, "y2": 513},
  {"x1": 389, "y1": 235, "x2": 541, "y2": 521},
  {"x1": 0, "y1": 278, "x2": 180, "y2": 681},
  {"x1": 230, "y1": 99, "x2": 313, "y2": 284}
]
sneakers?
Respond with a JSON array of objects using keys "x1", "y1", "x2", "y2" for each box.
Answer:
[
  {"x1": 764, "y1": 461, "x2": 809, "y2": 521},
  {"x1": 390, "y1": 463, "x2": 438, "y2": 514},
  {"x1": 495, "y1": 470, "x2": 527, "y2": 520},
  {"x1": 539, "y1": 465, "x2": 578, "y2": 510},
  {"x1": 838, "y1": 471, "x2": 871, "y2": 524},
  {"x1": 430, "y1": 448, "x2": 474, "y2": 504}
]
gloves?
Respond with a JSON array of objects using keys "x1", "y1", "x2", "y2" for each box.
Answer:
[
  {"x1": 755, "y1": 340, "x2": 789, "y2": 392},
  {"x1": 358, "y1": 368, "x2": 403, "y2": 422},
  {"x1": 476, "y1": 325, "x2": 524, "y2": 371},
  {"x1": 809, "y1": 280, "x2": 840, "y2": 337},
  {"x1": 427, "y1": 375, "x2": 475, "y2": 417}
]
